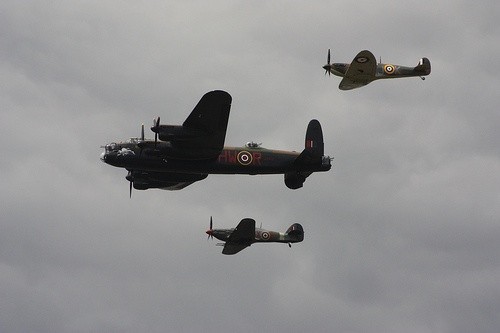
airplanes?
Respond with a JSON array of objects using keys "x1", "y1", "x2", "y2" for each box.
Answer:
[
  {"x1": 321, "y1": 49, "x2": 431, "y2": 91},
  {"x1": 98, "y1": 88, "x2": 336, "y2": 200},
  {"x1": 204, "y1": 215, "x2": 305, "y2": 255}
]
